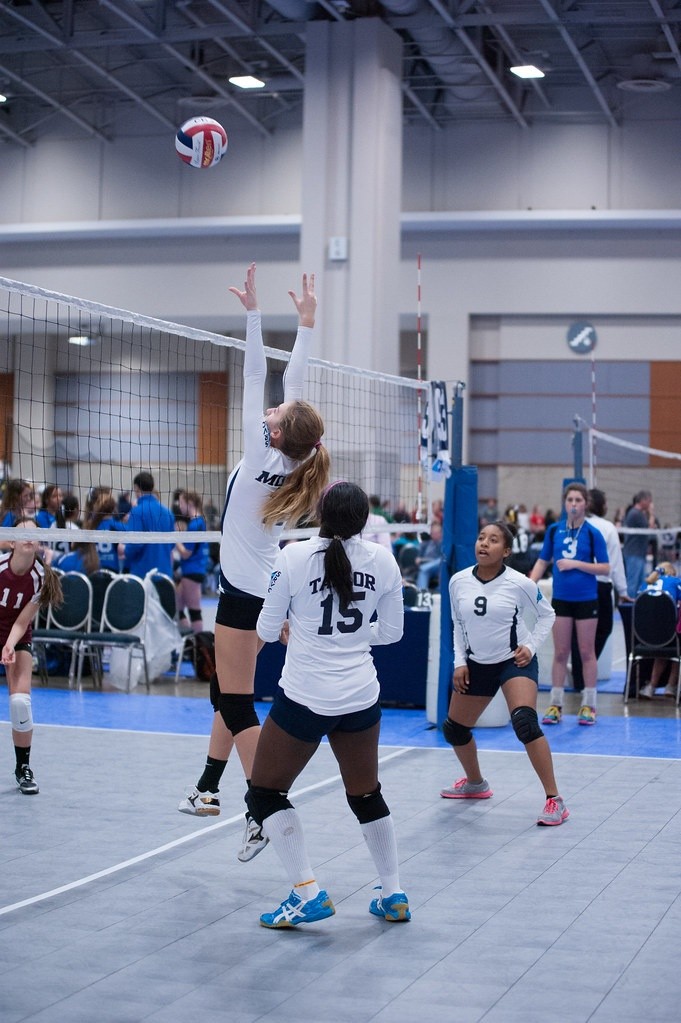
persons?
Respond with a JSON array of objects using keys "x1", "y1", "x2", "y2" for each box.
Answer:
[
  {"x1": 570, "y1": 487, "x2": 634, "y2": 690},
  {"x1": 612, "y1": 490, "x2": 681, "y2": 700},
  {"x1": 178, "y1": 261, "x2": 332, "y2": 862},
  {"x1": 529, "y1": 484, "x2": 610, "y2": 725},
  {"x1": 352, "y1": 491, "x2": 444, "y2": 600},
  {"x1": 440, "y1": 523, "x2": 571, "y2": 827},
  {"x1": 0, "y1": 472, "x2": 212, "y2": 670},
  {"x1": 245, "y1": 479, "x2": 413, "y2": 927},
  {"x1": 476, "y1": 496, "x2": 556, "y2": 558},
  {"x1": 0, "y1": 518, "x2": 64, "y2": 795}
]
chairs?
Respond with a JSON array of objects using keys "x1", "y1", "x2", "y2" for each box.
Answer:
[
  {"x1": 30, "y1": 566, "x2": 200, "y2": 694},
  {"x1": 400, "y1": 541, "x2": 419, "y2": 584},
  {"x1": 623, "y1": 588, "x2": 681, "y2": 707}
]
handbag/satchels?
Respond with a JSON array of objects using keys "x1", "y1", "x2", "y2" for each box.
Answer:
[{"x1": 187, "y1": 631, "x2": 217, "y2": 681}]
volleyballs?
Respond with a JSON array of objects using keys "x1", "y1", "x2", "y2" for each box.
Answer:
[{"x1": 175, "y1": 115, "x2": 228, "y2": 170}]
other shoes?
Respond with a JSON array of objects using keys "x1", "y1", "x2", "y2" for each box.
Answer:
[
  {"x1": 663, "y1": 685, "x2": 676, "y2": 696},
  {"x1": 638, "y1": 684, "x2": 654, "y2": 698}
]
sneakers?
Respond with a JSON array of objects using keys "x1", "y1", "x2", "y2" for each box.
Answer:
[
  {"x1": 439, "y1": 778, "x2": 493, "y2": 799},
  {"x1": 536, "y1": 795, "x2": 569, "y2": 825},
  {"x1": 177, "y1": 785, "x2": 223, "y2": 817},
  {"x1": 577, "y1": 704, "x2": 596, "y2": 725},
  {"x1": 368, "y1": 892, "x2": 412, "y2": 922},
  {"x1": 14, "y1": 765, "x2": 42, "y2": 796},
  {"x1": 257, "y1": 890, "x2": 337, "y2": 928},
  {"x1": 237, "y1": 810, "x2": 269, "y2": 862},
  {"x1": 542, "y1": 705, "x2": 563, "y2": 724}
]
